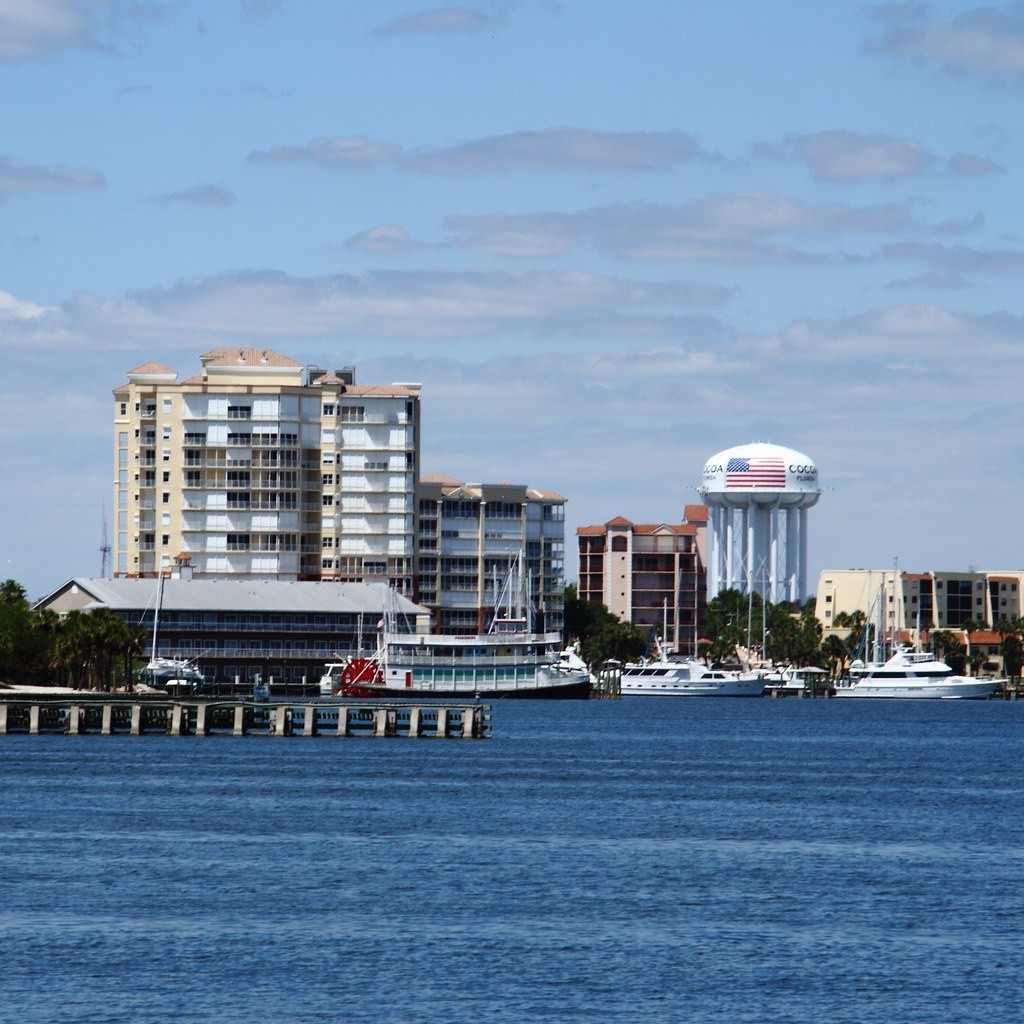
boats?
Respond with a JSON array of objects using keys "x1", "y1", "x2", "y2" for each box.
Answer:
[
  {"x1": 829, "y1": 651, "x2": 1008, "y2": 698},
  {"x1": 603, "y1": 660, "x2": 771, "y2": 698},
  {"x1": 319, "y1": 549, "x2": 603, "y2": 700},
  {"x1": 135, "y1": 658, "x2": 206, "y2": 696}
]
